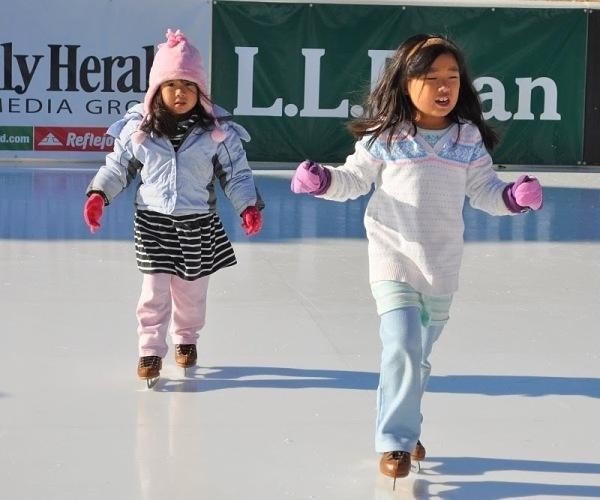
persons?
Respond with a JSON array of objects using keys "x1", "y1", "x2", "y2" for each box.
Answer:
[
  {"x1": 290, "y1": 35, "x2": 543, "y2": 479},
  {"x1": 84, "y1": 29, "x2": 265, "y2": 378}
]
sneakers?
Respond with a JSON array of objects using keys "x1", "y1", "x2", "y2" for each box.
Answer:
[
  {"x1": 380, "y1": 451, "x2": 411, "y2": 479},
  {"x1": 138, "y1": 356, "x2": 162, "y2": 379},
  {"x1": 410, "y1": 440, "x2": 425, "y2": 460},
  {"x1": 175, "y1": 344, "x2": 197, "y2": 368}
]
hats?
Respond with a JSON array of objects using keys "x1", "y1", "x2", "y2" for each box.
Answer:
[{"x1": 132, "y1": 28, "x2": 226, "y2": 143}]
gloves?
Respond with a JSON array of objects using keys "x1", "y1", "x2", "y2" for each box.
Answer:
[
  {"x1": 290, "y1": 160, "x2": 331, "y2": 195},
  {"x1": 83, "y1": 193, "x2": 105, "y2": 232},
  {"x1": 502, "y1": 174, "x2": 543, "y2": 213},
  {"x1": 240, "y1": 206, "x2": 262, "y2": 236}
]
